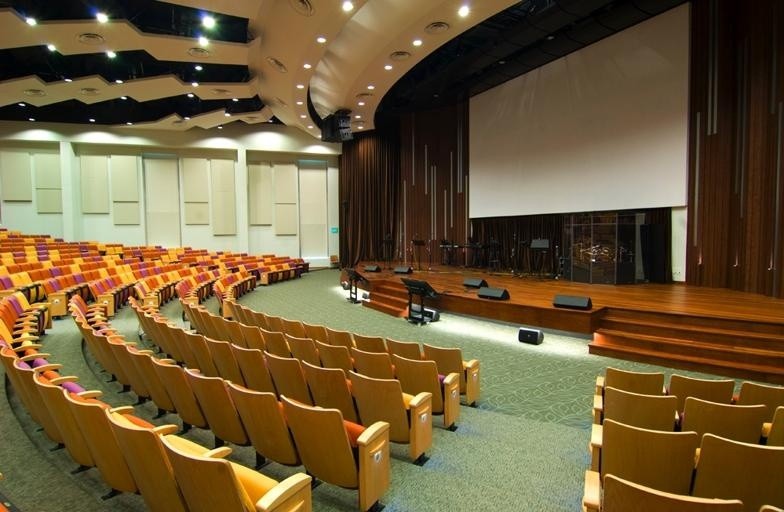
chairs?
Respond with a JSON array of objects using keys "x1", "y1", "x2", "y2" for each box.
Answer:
[{"x1": 583, "y1": 366, "x2": 784, "y2": 512}]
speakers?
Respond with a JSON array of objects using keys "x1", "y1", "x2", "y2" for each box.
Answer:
[
  {"x1": 365, "y1": 265, "x2": 381, "y2": 272},
  {"x1": 423, "y1": 307, "x2": 439, "y2": 322},
  {"x1": 553, "y1": 294, "x2": 592, "y2": 310},
  {"x1": 478, "y1": 286, "x2": 510, "y2": 299},
  {"x1": 518, "y1": 327, "x2": 544, "y2": 344},
  {"x1": 321, "y1": 114, "x2": 354, "y2": 144},
  {"x1": 393, "y1": 266, "x2": 413, "y2": 274},
  {"x1": 342, "y1": 281, "x2": 350, "y2": 290},
  {"x1": 463, "y1": 278, "x2": 488, "y2": 288},
  {"x1": 362, "y1": 291, "x2": 370, "y2": 299}
]
